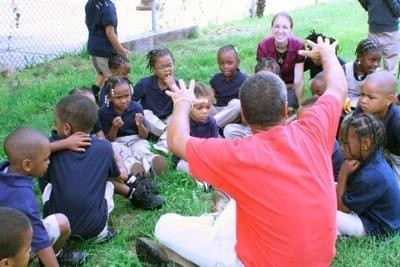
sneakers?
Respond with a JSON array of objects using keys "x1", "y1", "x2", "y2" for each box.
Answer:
[
  {"x1": 131, "y1": 178, "x2": 164, "y2": 210},
  {"x1": 136, "y1": 238, "x2": 197, "y2": 267},
  {"x1": 38, "y1": 249, "x2": 84, "y2": 267}
]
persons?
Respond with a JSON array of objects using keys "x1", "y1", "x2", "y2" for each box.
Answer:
[
  {"x1": 0, "y1": 29, "x2": 400, "y2": 267},
  {"x1": 358, "y1": 0, "x2": 400, "y2": 80},
  {"x1": 255, "y1": 11, "x2": 307, "y2": 117},
  {"x1": 85, "y1": 0, "x2": 131, "y2": 107}
]
